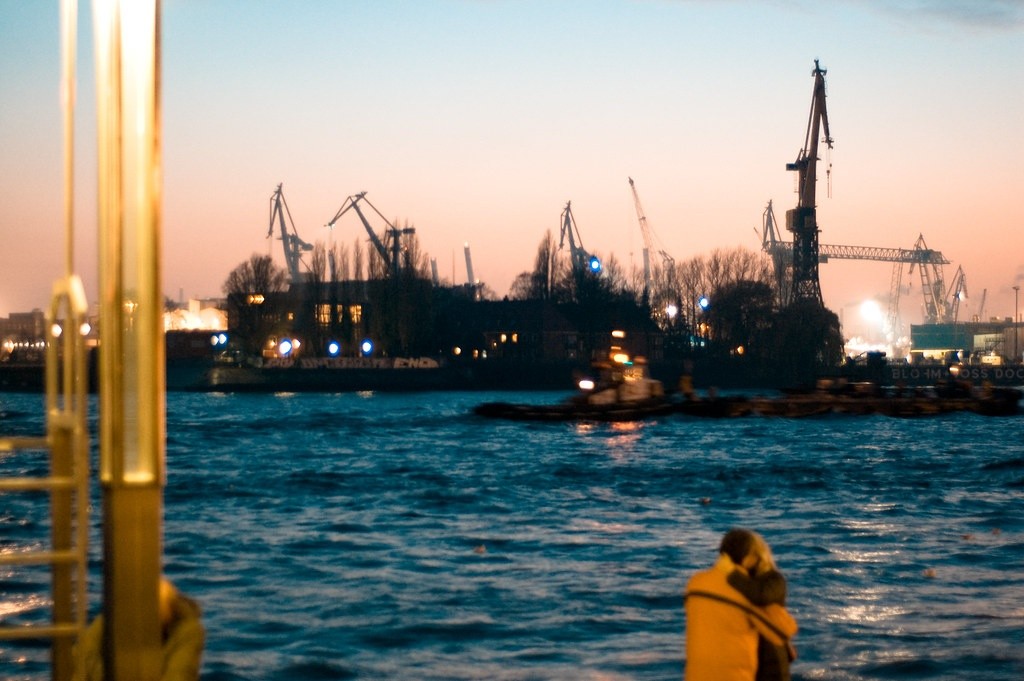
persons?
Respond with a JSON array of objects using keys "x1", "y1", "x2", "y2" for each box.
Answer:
[
  {"x1": 683, "y1": 532, "x2": 797, "y2": 681},
  {"x1": 718, "y1": 532, "x2": 794, "y2": 681}
]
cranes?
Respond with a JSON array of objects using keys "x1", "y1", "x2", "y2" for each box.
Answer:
[
  {"x1": 944, "y1": 263, "x2": 968, "y2": 325},
  {"x1": 268, "y1": 182, "x2": 313, "y2": 287},
  {"x1": 329, "y1": 191, "x2": 401, "y2": 272},
  {"x1": 556, "y1": 202, "x2": 583, "y2": 271},
  {"x1": 628, "y1": 176, "x2": 665, "y2": 296},
  {"x1": 905, "y1": 235, "x2": 945, "y2": 323},
  {"x1": 761, "y1": 201, "x2": 949, "y2": 272},
  {"x1": 786, "y1": 58, "x2": 835, "y2": 305}
]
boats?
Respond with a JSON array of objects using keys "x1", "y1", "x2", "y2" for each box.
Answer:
[
  {"x1": 940, "y1": 377, "x2": 976, "y2": 410},
  {"x1": 467, "y1": 328, "x2": 747, "y2": 421},
  {"x1": 972, "y1": 386, "x2": 1024, "y2": 417},
  {"x1": 752, "y1": 365, "x2": 874, "y2": 415},
  {"x1": 885, "y1": 379, "x2": 940, "y2": 416}
]
